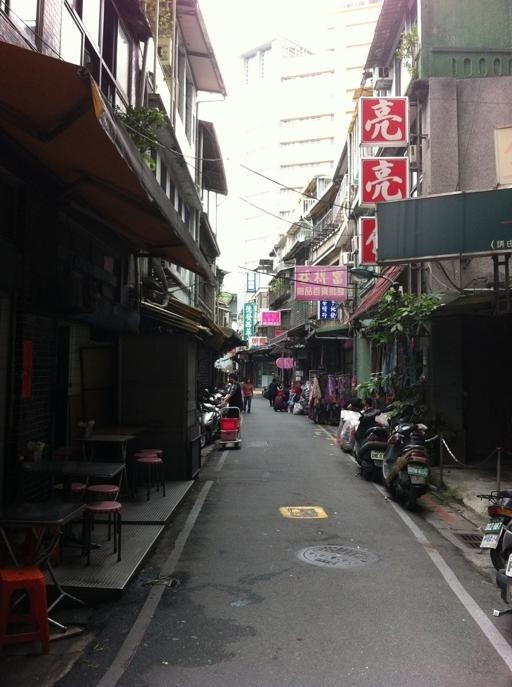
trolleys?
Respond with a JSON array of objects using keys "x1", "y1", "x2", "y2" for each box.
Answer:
[{"x1": 220, "y1": 407, "x2": 243, "y2": 448}]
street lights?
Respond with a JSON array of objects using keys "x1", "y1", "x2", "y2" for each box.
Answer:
[{"x1": 351, "y1": 268, "x2": 394, "y2": 284}]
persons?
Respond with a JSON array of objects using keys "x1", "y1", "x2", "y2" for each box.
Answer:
[
  {"x1": 290, "y1": 381, "x2": 303, "y2": 408},
  {"x1": 270, "y1": 390, "x2": 289, "y2": 413},
  {"x1": 242, "y1": 378, "x2": 255, "y2": 414},
  {"x1": 224, "y1": 373, "x2": 244, "y2": 414},
  {"x1": 267, "y1": 378, "x2": 277, "y2": 407}
]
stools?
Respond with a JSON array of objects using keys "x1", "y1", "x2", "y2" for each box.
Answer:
[{"x1": 0, "y1": 450, "x2": 166, "y2": 653}]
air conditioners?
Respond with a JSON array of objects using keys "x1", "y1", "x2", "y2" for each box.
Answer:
[{"x1": 372, "y1": 66, "x2": 390, "y2": 89}]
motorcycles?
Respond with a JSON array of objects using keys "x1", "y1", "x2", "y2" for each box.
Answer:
[
  {"x1": 476, "y1": 489, "x2": 512, "y2": 620},
  {"x1": 345, "y1": 396, "x2": 437, "y2": 504},
  {"x1": 198, "y1": 389, "x2": 227, "y2": 448}
]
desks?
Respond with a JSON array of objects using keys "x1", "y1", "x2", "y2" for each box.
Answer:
[{"x1": 74, "y1": 434, "x2": 136, "y2": 502}]
